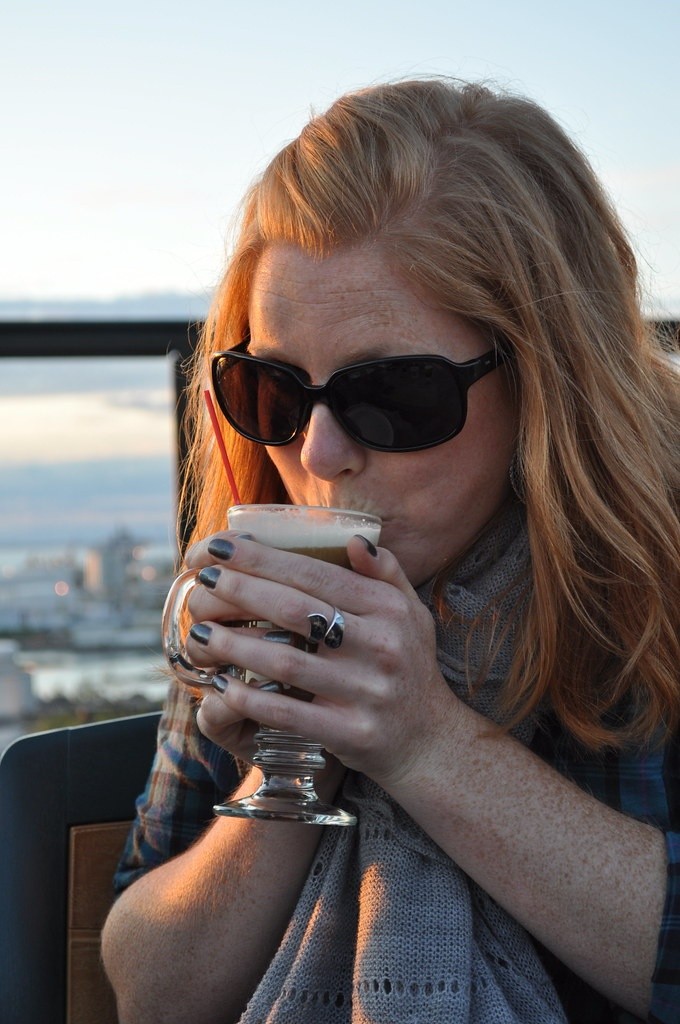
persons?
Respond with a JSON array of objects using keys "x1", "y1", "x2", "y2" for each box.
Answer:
[{"x1": 101, "y1": 81, "x2": 680, "y2": 1024}]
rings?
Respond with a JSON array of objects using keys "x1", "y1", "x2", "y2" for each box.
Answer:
[{"x1": 307, "y1": 605, "x2": 345, "y2": 649}]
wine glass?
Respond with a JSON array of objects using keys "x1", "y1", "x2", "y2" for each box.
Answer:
[{"x1": 160, "y1": 502, "x2": 384, "y2": 826}]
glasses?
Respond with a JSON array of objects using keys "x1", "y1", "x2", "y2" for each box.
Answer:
[{"x1": 210, "y1": 334, "x2": 511, "y2": 452}]
